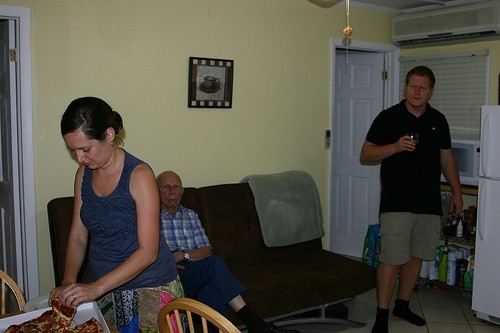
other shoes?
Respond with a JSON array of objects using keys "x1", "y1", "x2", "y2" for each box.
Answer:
[{"x1": 248, "y1": 323, "x2": 300, "y2": 333}]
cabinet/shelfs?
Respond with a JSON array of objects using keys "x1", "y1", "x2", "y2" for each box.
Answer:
[{"x1": 418, "y1": 183, "x2": 478, "y2": 296}]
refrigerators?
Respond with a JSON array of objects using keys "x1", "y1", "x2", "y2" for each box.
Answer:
[{"x1": 471, "y1": 105, "x2": 500, "y2": 324}]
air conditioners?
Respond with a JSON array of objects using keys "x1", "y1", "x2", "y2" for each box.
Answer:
[{"x1": 390, "y1": 1, "x2": 500, "y2": 48}]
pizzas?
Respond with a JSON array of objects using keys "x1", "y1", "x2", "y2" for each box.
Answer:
[{"x1": 2, "y1": 297, "x2": 104, "y2": 333}]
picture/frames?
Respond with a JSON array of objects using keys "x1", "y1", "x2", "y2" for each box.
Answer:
[{"x1": 188, "y1": 56, "x2": 234, "y2": 109}]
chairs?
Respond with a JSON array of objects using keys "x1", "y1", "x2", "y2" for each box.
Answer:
[{"x1": 157, "y1": 296, "x2": 242, "y2": 333}]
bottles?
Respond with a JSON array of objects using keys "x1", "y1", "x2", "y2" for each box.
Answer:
[
  {"x1": 446, "y1": 205, "x2": 477, "y2": 237},
  {"x1": 414, "y1": 240, "x2": 475, "y2": 290}
]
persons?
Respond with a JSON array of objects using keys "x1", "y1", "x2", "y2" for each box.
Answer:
[
  {"x1": 360, "y1": 65, "x2": 463, "y2": 333},
  {"x1": 156, "y1": 170, "x2": 300, "y2": 333},
  {"x1": 49, "y1": 96, "x2": 189, "y2": 333}
]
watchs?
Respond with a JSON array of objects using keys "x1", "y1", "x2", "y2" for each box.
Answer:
[{"x1": 182, "y1": 250, "x2": 190, "y2": 261}]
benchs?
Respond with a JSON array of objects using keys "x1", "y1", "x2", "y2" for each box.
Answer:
[{"x1": 47, "y1": 171, "x2": 377, "y2": 333}]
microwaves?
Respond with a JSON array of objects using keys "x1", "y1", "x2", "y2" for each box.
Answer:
[{"x1": 441, "y1": 139, "x2": 481, "y2": 187}]
